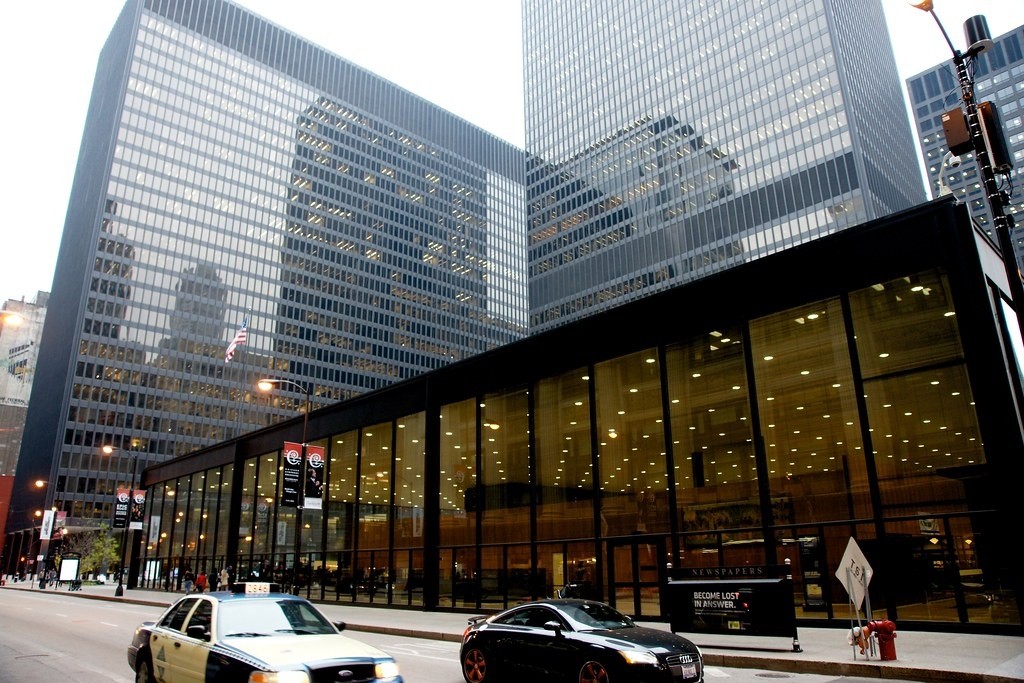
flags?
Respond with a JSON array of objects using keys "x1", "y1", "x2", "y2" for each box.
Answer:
[
  {"x1": 301, "y1": 445, "x2": 326, "y2": 510},
  {"x1": 278, "y1": 521, "x2": 287, "y2": 547},
  {"x1": 254, "y1": 502, "x2": 270, "y2": 536},
  {"x1": 224, "y1": 315, "x2": 247, "y2": 364},
  {"x1": 112, "y1": 488, "x2": 130, "y2": 531},
  {"x1": 141, "y1": 515, "x2": 148, "y2": 541},
  {"x1": 238, "y1": 499, "x2": 251, "y2": 539},
  {"x1": 280, "y1": 441, "x2": 303, "y2": 507},
  {"x1": 129, "y1": 489, "x2": 146, "y2": 531},
  {"x1": 39, "y1": 509, "x2": 53, "y2": 540},
  {"x1": 52, "y1": 510, "x2": 68, "y2": 540},
  {"x1": 149, "y1": 515, "x2": 160, "y2": 544}
]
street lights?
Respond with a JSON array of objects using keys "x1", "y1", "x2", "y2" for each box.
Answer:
[
  {"x1": 35, "y1": 480, "x2": 61, "y2": 588},
  {"x1": 257, "y1": 377, "x2": 311, "y2": 596},
  {"x1": 908, "y1": 0, "x2": 1024, "y2": 344},
  {"x1": 103, "y1": 444, "x2": 138, "y2": 598}
]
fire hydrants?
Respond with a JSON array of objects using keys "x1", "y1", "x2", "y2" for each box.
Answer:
[{"x1": 867, "y1": 618, "x2": 897, "y2": 660}]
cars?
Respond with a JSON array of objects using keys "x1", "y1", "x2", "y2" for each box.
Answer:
[
  {"x1": 126, "y1": 581, "x2": 404, "y2": 683},
  {"x1": 460, "y1": 599, "x2": 704, "y2": 683}
]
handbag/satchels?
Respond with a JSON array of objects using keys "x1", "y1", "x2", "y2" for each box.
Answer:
[{"x1": 196, "y1": 583, "x2": 202, "y2": 588}]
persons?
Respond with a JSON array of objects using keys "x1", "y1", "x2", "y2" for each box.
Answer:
[
  {"x1": 255, "y1": 559, "x2": 385, "y2": 588},
  {"x1": 184, "y1": 568, "x2": 194, "y2": 595},
  {"x1": 14, "y1": 568, "x2": 82, "y2": 591},
  {"x1": 226, "y1": 565, "x2": 236, "y2": 590},
  {"x1": 220, "y1": 569, "x2": 230, "y2": 591},
  {"x1": 208, "y1": 567, "x2": 219, "y2": 592},
  {"x1": 217, "y1": 577, "x2": 222, "y2": 591},
  {"x1": 194, "y1": 570, "x2": 207, "y2": 593}
]
